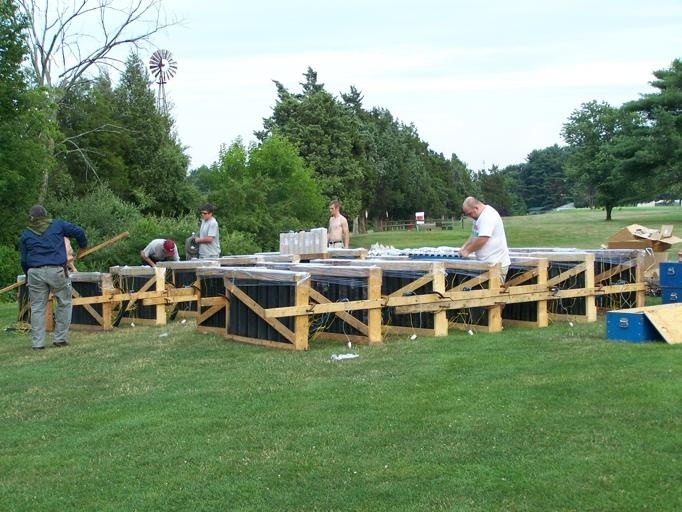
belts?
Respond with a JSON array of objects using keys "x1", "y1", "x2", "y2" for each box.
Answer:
[{"x1": 329, "y1": 241, "x2": 341, "y2": 244}]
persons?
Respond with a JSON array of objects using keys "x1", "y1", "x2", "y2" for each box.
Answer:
[
  {"x1": 193, "y1": 204, "x2": 221, "y2": 258},
  {"x1": 326, "y1": 200, "x2": 349, "y2": 249},
  {"x1": 141, "y1": 238, "x2": 180, "y2": 267},
  {"x1": 21, "y1": 206, "x2": 88, "y2": 349},
  {"x1": 458, "y1": 196, "x2": 511, "y2": 325}
]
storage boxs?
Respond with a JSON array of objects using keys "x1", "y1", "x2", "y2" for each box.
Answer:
[{"x1": 606, "y1": 262, "x2": 682, "y2": 345}]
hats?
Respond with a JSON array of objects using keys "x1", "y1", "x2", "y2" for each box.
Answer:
[{"x1": 164, "y1": 240, "x2": 174, "y2": 256}]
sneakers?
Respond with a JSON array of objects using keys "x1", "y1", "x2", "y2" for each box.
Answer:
[
  {"x1": 32, "y1": 345, "x2": 44, "y2": 350},
  {"x1": 55, "y1": 342, "x2": 69, "y2": 347}
]
